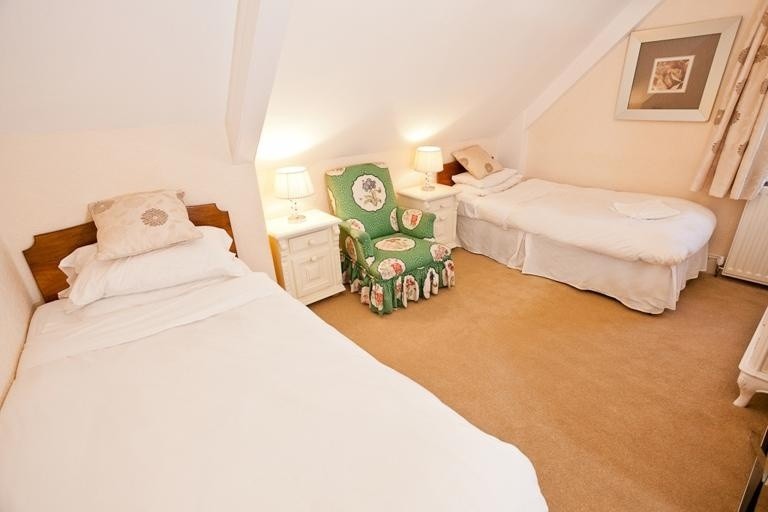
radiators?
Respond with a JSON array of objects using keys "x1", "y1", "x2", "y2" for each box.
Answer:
[{"x1": 721, "y1": 184, "x2": 768, "y2": 285}]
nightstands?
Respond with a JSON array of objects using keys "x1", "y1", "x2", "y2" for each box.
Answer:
[
  {"x1": 397, "y1": 183, "x2": 462, "y2": 253},
  {"x1": 266, "y1": 209, "x2": 347, "y2": 307}
]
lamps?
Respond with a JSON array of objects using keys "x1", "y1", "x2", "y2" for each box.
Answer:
[
  {"x1": 275, "y1": 165, "x2": 315, "y2": 222},
  {"x1": 414, "y1": 145, "x2": 444, "y2": 191}
]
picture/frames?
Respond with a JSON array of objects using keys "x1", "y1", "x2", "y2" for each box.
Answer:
[{"x1": 612, "y1": 15, "x2": 744, "y2": 124}]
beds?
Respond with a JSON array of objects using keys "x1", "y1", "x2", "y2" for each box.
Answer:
[
  {"x1": 436, "y1": 160, "x2": 718, "y2": 316},
  {"x1": 0, "y1": 204, "x2": 551, "y2": 512}
]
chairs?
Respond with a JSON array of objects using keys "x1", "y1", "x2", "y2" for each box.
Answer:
[{"x1": 322, "y1": 160, "x2": 455, "y2": 314}]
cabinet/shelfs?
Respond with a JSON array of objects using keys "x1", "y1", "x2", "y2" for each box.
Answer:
[{"x1": 733, "y1": 306, "x2": 768, "y2": 409}]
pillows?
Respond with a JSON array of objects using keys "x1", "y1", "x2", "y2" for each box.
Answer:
[
  {"x1": 450, "y1": 143, "x2": 504, "y2": 180},
  {"x1": 57, "y1": 224, "x2": 244, "y2": 312},
  {"x1": 449, "y1": 166, "x2": 517, "y2": 189},
  {"x1": 57, "y1": 255, "x2": 253, "y2": 314},
  {"x1": 452, "y1": 173, "x2": 523, "y2": 197},
  {"x1": 87, "y1": 189, "x2": 203, "y2": 262}
]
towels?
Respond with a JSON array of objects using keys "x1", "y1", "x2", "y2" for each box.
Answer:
[{"x1": 610, "y1": 199, "x2": 680, "y2": 222}]
